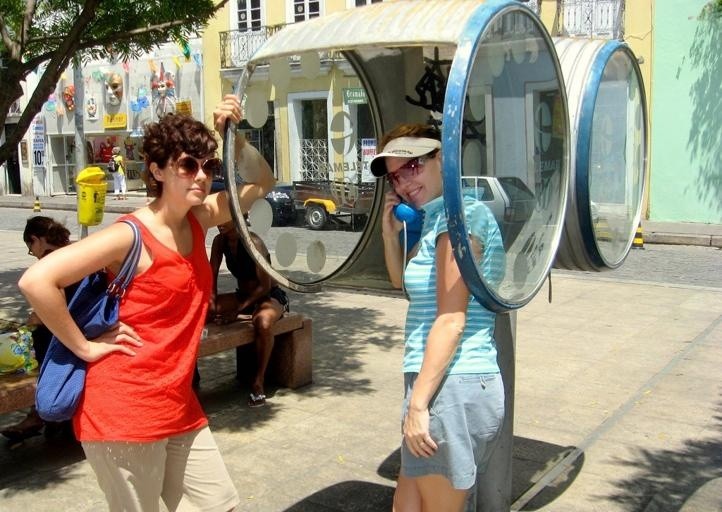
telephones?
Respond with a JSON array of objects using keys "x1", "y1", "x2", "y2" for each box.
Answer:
[{"x1": 393, "y1": 203, "x2": 419, "y2": 223}]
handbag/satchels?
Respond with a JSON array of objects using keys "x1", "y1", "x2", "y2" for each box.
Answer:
[{"x1": 33, "y1": 219, "x2": 143, "y2": 423}]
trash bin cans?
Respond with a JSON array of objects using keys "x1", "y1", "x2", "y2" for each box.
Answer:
[{"x1": 76, "y1": 165, "x2": 108, "y2": 225}]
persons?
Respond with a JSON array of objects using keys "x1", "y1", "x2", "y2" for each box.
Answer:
[
  {"x1": 193, "y1": 213, "x2": 290, "y2": 410},
  {"x1": 16, "y1": 95, "x2": 277, "y2": 512},
  {"x1": 1, "y1": 216, "x2": 82, "y2": 458},
  {"x1": 367, "y1": 121, "x2": 508, "y2": 512},
  {"x1": 87, "y1": 136, "x2": 144, "y2": 179},
  {"x1": 112, "y1": 146, "x2": 128, "y2": 200}
]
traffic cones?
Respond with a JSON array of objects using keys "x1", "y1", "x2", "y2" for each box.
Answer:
[
  {"x1": 631, "y1": 221, "x2": 645, "y2": 249},
  {"x1": 597, "y1": 216, "x2": 611, "y2": 241},
  {"x1": 33, "y1": 196, "x2": 41, "y2": 212}
]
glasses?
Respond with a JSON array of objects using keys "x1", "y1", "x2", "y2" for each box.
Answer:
[
  {"x1": 384, "y1": 149, "x2": 438, "y2": 189},
  {"x1": 162, "y1": 156, "x2": 223, "y2": 180}
]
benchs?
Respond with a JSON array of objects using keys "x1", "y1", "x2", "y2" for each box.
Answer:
[{"x1": 2, "y1": 315, "x2": 316, "y2": 460}]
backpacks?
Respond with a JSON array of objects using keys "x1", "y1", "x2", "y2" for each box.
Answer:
[{"x1": 107, "y1": 156, "x2": 121, "y2": 173}]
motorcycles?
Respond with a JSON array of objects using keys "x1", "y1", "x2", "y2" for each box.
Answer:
[{"x1": 292, "y1": 180, "x2": 375, "y2": 230}]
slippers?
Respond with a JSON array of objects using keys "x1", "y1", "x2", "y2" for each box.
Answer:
[
  {"x1": 0, "y1": 420, "x2": 70, "y2": 441},
  {"x1": 247, "y1": 391, "x2": 267, "y2": 409}
]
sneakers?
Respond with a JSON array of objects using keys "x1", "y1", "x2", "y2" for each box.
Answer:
[{"x1": 113, "y1": 195, "x2": 129, "y2": 200}]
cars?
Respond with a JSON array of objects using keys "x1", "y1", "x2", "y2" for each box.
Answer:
[
  {"x1": 235, "y1": 165, "x2": 297, "y2": 227},
  {"x1": 461, "y1": 176, "x2": 536, "y2": 253}
]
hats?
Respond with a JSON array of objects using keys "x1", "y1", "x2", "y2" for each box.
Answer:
[
  {"x1": 111, "y1": 146, "x2": 121, "y2": 155},
  {"x1": 370, "y1": 136, "x2": 442, "y2": 178}
]
